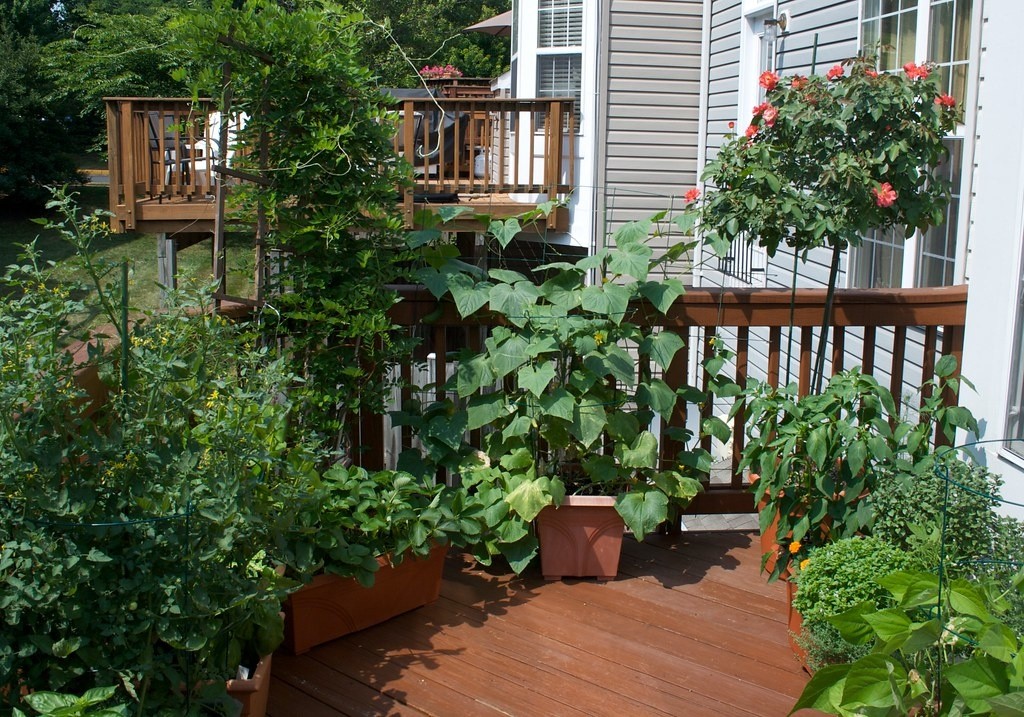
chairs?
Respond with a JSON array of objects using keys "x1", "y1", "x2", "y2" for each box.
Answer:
[
  {"x1": 367, "y1": 112, "x2": 423, "y2": 197},
  {"x1": 137, "y1": 112, "x2": 218, "y2": 205}
]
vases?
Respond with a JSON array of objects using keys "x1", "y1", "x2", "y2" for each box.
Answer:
[
  {"x1": 531, "y1": 462, "x2": 634, "y2": 583},
  {"x1": 420, "y1": 72, "x2": 458, "y2": 93},
  {"x1": 178, "y1": 560, "x2": 287, "y2": 717},
  {"x1": 747, "y1": 456, "x2": 944, "y2": 717}
]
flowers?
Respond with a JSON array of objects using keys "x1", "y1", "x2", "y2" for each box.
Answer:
[
  {"x1": 419, "y1": 65, "x2": 463, "y2": 78},
  {"x1": 727, "y1": 349, "x2": 1024, "y2": 717},
  {"x1": 681, "y1": 38, "x2": 965, "y2": 255},
  {"x1": 0, "y1": 181, "x2": 359, "y2": 717},
  {"x1": 394, "y1": 198, "x2": 742, "y2": 577}
]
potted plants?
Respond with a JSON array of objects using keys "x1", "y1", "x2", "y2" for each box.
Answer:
[{"x1": 34, "y1": 0, "x2": 455, "y2": 657}]
